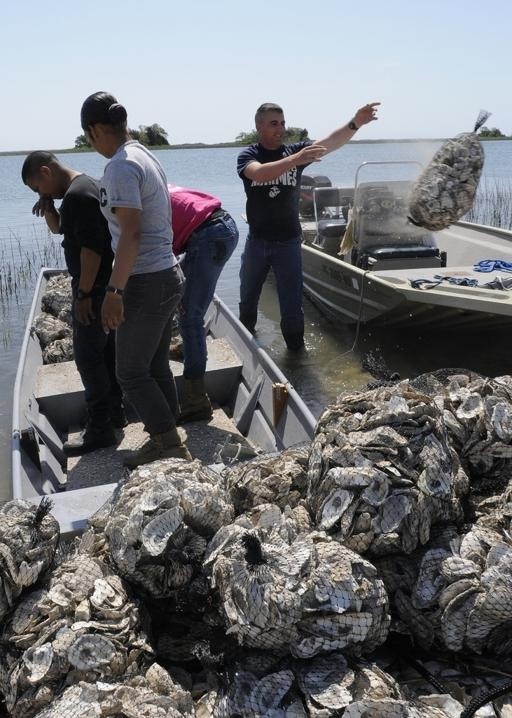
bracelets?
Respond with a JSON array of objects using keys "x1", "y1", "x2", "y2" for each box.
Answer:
[{"x1": 104, "y1": 284, "x2": 126, "y2": 297}]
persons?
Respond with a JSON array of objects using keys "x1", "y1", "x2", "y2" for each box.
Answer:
[
  {"x1": 20, "y1": 151, "x2": 129, "y2": 457},
  {"x1": 167, "y1": 181, "x2": 240, "y2": 426},
  {"x1": 80, "y1": 89, "x2": 194, "y2": 467},
  {"x1": 236, "y1": 100, "x2": 383, "y2": 349}
]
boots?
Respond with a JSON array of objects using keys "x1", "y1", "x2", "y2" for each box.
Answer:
[
  {"x1": 123, "y1": 427, "x2": 194, "y2": 471},
  {"x1": 176, "y1": 375, "x2": 213, "y2": 425},
  {"x1": 168, "y1": 337, "x2": 185, "y2": 359},
  {"x1": 62, "y1": 398, "x2": 128, "y2": 457}
]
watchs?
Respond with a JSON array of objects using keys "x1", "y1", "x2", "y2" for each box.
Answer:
[
  {"x1": 347, "y1": 120, "x2": 360, "y2": 132},
  {"x1": 75, "y1": 289, "x2": 93, "y2": 301}
]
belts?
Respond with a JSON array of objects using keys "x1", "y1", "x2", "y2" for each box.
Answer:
[{"x1": 189, "y1": 214, "x2": 231, "y2": 238}]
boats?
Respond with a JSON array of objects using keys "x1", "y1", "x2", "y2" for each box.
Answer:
[
  {"x1": 241, "y1": 162, "x2": 512, "y2": 336},
  {"x1": 13, "y1": 268, "x2": 323, "y2": 548}
]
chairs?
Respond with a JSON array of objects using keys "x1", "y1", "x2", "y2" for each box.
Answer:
[{"x1": 313, "y1": 180, "x2": 439, "y2": 257}]
